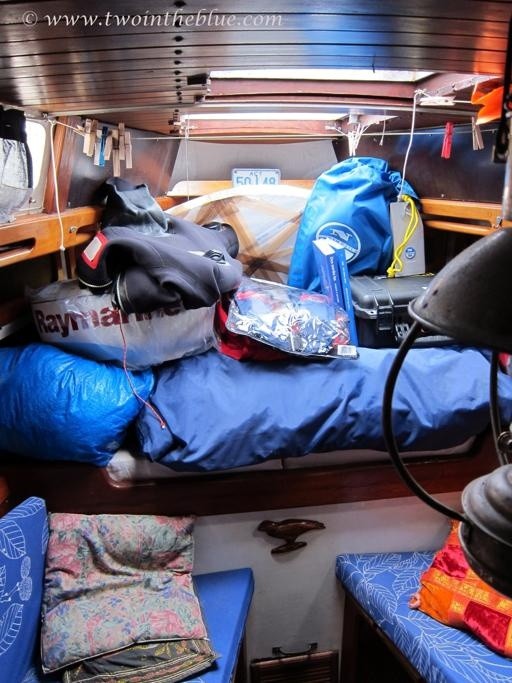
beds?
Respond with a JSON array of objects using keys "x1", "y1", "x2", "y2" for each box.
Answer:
[{"x1": 12, "y1": 447, "x2": 511, "y2": 682}]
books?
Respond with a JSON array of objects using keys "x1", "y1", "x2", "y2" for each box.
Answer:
[
  {"x1": 324, "y1": 239, "x2": 359, "y2": 347},
  {"x1": 312, "y1": 238, "x2": 344, "y2": 309}
]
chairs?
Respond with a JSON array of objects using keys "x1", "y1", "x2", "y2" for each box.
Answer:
[
  {"x1": 1, "y1": 493, "x2": 255, "y2": 682},
  {"x1": 333, "y1": 537, "x2": 511, "y2": 682}
]
